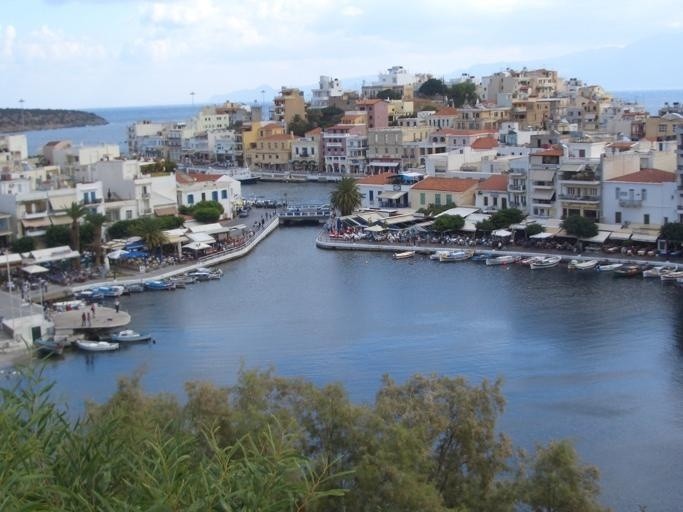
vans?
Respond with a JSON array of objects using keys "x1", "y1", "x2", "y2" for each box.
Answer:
[{"x1": 316, "y1": 203, "x2": 330, "y2": 215}]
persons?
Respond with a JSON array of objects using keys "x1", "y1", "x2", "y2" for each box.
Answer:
[
  {"x1": 324, "y1": 216, "x2": 683, "y2": 260},
  {"x1": 0, "y1": 196, "x2": 287, "y2": 329}
]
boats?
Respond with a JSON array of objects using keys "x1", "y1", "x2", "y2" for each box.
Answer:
[
  {"x1": 428, "y1": 247, "x2": 683, "y2": 279},
  {"x1": 49, "y1": 266, "x2": 225, "y2": 314},
  {"x1": 33, "y1": 333, "x2": 66, "y2": 356},
  {"x1": 75, "y1": 339, "x2": 119, "y2": 352},
  {"x1": 204, "y1": 166, "x2": 261, "y2": 185},
  {"x1": 104, "y1": 329, "x2": 152, "y2": 342},
  {"x1": 392, "y1": 250, "x2": 415, "y2": 260}
]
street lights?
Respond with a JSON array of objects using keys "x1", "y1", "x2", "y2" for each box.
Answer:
[
  {"x1": 18, "y1": 98, "x2": 25, "y2": 135},
  {"x1": 189, "y1": 91, "x2": 196, "y2": 118},
  {"x1": 35, "y1": 278, "x2": 49, "y2": 314},
  {"x1": 260, "y1": 90, "x2": 266, "y2": 104},
  {"x1": 3, "y1": 248, "x2": 15, "y2": 330}
]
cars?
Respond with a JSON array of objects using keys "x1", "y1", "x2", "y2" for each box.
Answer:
[
  {"x1": 239, "y1": 199, "x2": 288, "y2": 217},
  {"x1": 286, "y1": 209, "x2": 303, "y2": 215}
]
